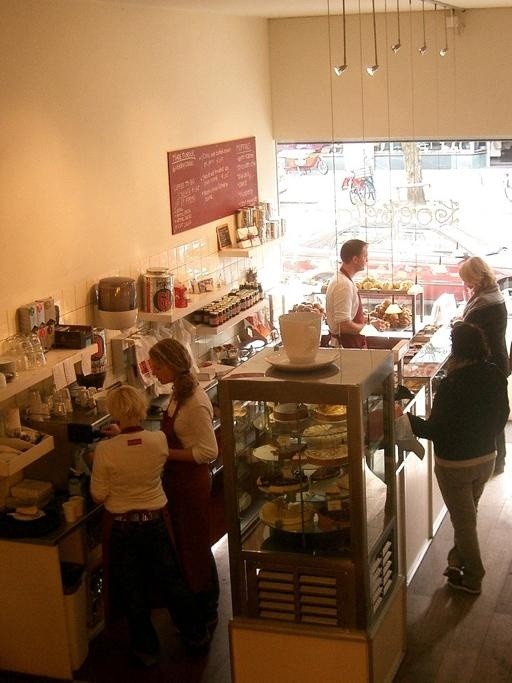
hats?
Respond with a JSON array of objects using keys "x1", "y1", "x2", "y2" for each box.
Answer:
[{"x1": 394, "y1": 414, "x2": 425, "y2": 459}]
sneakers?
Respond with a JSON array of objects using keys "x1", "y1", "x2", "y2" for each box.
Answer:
[
  {"x1": 495, "y1": 457, "x2": 505, "y2": 472},
  {"x1": 448, "y1": 576, "x2": 482, "y2": 594},
  {"x1": 443, "y1": 559, "x2": 461, "y2": 578}
]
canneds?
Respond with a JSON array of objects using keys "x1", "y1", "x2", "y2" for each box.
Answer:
[{"x1": 193, "y1": 288, "x2": 260, "y2": 328}]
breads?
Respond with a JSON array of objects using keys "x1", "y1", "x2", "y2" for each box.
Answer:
[
  {"x1": 354, "y1": 276, "x2": 415, "y2": 291},
  {"x1": 288, "y1": 301, "x2": 326, "y2": 321},
  {"x1": 367, "y1": 299, "x2": 413, "y2": 333}
]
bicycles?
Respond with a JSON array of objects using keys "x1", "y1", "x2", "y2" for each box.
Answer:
[{"x1": 346, "y1": 159, "x2": 380, "y2": 207}]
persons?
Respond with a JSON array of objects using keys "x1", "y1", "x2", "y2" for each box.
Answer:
[
  {"x1": 458, "y1": 256, "x2": 512, "y2": 476},
  {"x1": 325, "y1": 239, "x2": 368, "y2": 349},
  {"x1": 148, "y1": 337, "x2": 220, "y2": 646},
  {"x1": 83, "y1": 385, "x2": 209, "y2": 667},
  {"x1": 408, "y1": 322, "x2": 510, "y2": 595}
]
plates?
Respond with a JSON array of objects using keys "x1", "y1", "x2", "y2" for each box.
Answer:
[
  {"x1": 12, "y1": 509, "x2": 46, "y2": 521},
  {"x1": 264, "y1": 347, "x2": 342, "y2": 371}
]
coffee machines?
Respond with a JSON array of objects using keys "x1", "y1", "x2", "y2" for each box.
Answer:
[{"x1": 93, "y1": 277, "x2": 139, "y2": 331}]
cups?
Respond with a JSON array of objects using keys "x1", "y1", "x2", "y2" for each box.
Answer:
[
  {"x1": 24, "y1": 385, "x2": 109, "y2": 420},
  {"x1": 62, "y1": 496, "x2": 86, "y2": 522},
  {"x1": 0, "y1": 333, "x2": 47, "y2": 388}
]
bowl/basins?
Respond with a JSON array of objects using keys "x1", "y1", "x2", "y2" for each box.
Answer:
[{"x1": 279, "y1": 312, "x2": 321, "y2": 363}]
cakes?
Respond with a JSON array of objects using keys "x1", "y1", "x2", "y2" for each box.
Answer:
[
  {"x1": 309, "y1": 467, "x2": 340, "y2": 481},
  {"x1": 325, "y1": 486, "x2": 340, "y2": 494},
  {"x1": 304, "y1": 447, "x2": 349, "y2": 464},
  {"x1": 262, "y1": 502, "x2": 315, "y2": 524},
  {"x1": 317, "y1": 500, "x2": 350, "y2": 525},
  {"x1": 268, "y1": 403, "x2": 309, "y2": 421},
  {"x1": 313, "y1": 405, "x2": 347, "y2": 421},
  {"x1": 303, "y1": 424, "x2": 347, "y2": 445},
  {"x1": 257, "y1": 471, "x2": 309, "y2": 493},
  {"x1": 337, "y1": 473, "x2": 350, "y2": 490},
  {"x1": 271, "y1": 436, "x2": 306, "y2": 460}
]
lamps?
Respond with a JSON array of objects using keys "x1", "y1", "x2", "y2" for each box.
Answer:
[
  {"x1": 366, "y1": 0, "x2": 380, "y2": 76},
  {"x1": 419, "y1": 2, "x2": 428, "y2": 55},
  {"x1": 334, "y1": 0, "x2": 348, "y2": 75},
  {"x1": 392, "y1": 0, "x2": 402, "y2": 54},
  {"x1": 440, "y1": 7, "x2": 449, "y2": 56}
]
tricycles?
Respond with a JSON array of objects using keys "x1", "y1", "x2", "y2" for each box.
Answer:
[{"x1": 280, "y1": 148, "x2": 331, "y2": 176}]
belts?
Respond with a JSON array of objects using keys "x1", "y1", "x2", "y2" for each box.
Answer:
[{"x1": 113, "y1": 511, "x2": 160, "y2": 523}]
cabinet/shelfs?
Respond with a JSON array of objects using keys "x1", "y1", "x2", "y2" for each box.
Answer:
[
  {"x1": 368, "y1": 324, "x2": 449, "y2": 587},
  {"x1": 135, "y1": 285, "x2": 265, "y2": 335},
  {"x1": 320, "y1": 278, "x2": 425, "y2": 337},
  {"x1": 219, "y1": 346, "x2": 409, "y2": 683},
  {"x1": 1, "y1": 507, "x2": 108, "y2": 683},
  {"x1": 1, "y1": 343, "x2": 99, "y2": 479}
]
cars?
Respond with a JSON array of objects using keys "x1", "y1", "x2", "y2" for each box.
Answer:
[{"x1": 279, "y1": 218, "x2": 512, "y2": 314}]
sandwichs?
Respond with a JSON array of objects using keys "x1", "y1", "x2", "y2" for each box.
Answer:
[{"x1": 14, "y1": 505, "x2": 39, "y2": 518}]
